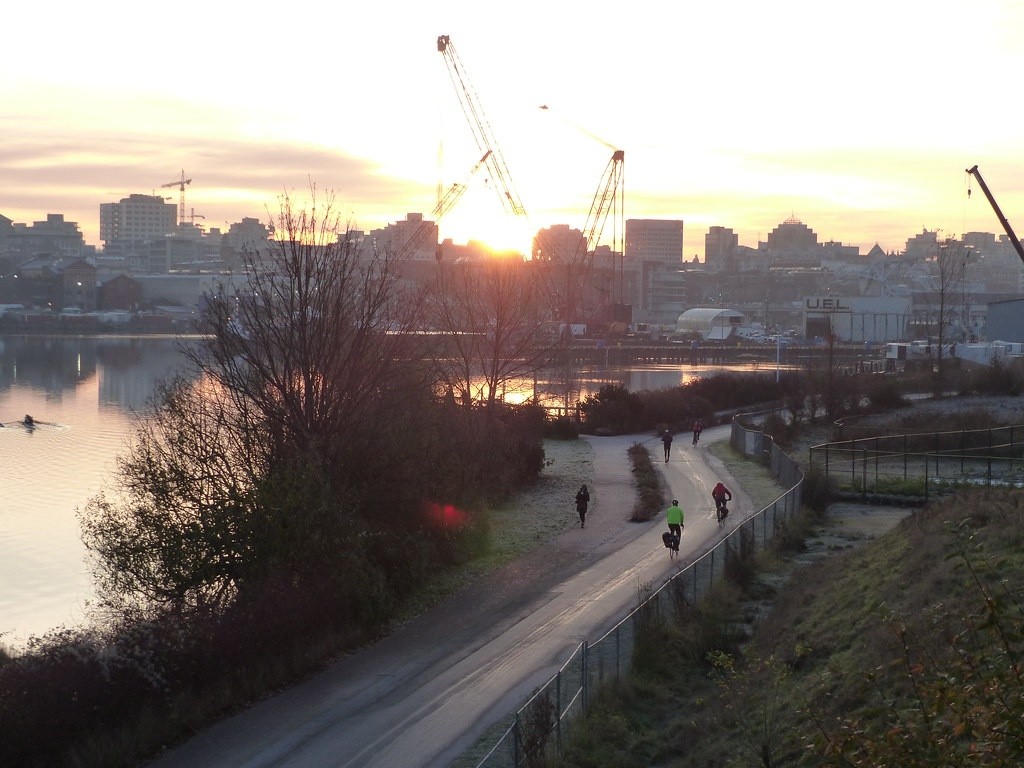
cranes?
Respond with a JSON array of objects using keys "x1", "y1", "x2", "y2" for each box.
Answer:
[
  {"x1": 161, "y1": 170, "x2": 191, "y2": 222},
  {"x1": 177, "y1": 208, "x2": 205, "y2": 226}
]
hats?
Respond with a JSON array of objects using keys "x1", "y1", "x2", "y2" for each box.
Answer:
[
  {"x1": 665, "y1": 430, "x2": 669, "y2": 432},
  {"x1": 672, "y1": 500, "x2": 678, "y2": 505}
]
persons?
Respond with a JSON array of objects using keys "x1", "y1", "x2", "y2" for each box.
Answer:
[
  {"x1": 692, "y1": 416, "x2": 704, "y2": 445},
  {"x1": 667, "y1": 500, "x2": 684, "y2": 553},
  {"x1": 576, "y1": 484, "x2": 590, "y2": 528},
  {"x1": 712, "y1": 482, "x2": 731, "y2": 524},
  {"x1": 661, "y1": 430, "x2": 673, "y2": 463}
]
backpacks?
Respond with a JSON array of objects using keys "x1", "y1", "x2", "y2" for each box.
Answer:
[{"x1": 715, "y1": 483, "x2": 724, "y2": 499}]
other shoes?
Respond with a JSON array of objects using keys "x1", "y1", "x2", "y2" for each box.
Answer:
[{"x1": 581, "y1": 524, "x2": 584, "y2": 528}]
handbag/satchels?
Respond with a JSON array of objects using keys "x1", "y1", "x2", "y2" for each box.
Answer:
[
  {"x1": 720, "y1": 507, "x2": 729, "y2": 520},
  {"x1": 575, "y1": 501, "x2": 578, "y2": 503},
  {"x1": 662, "y1": 532, "x2": 679, "y2": 551}
]
